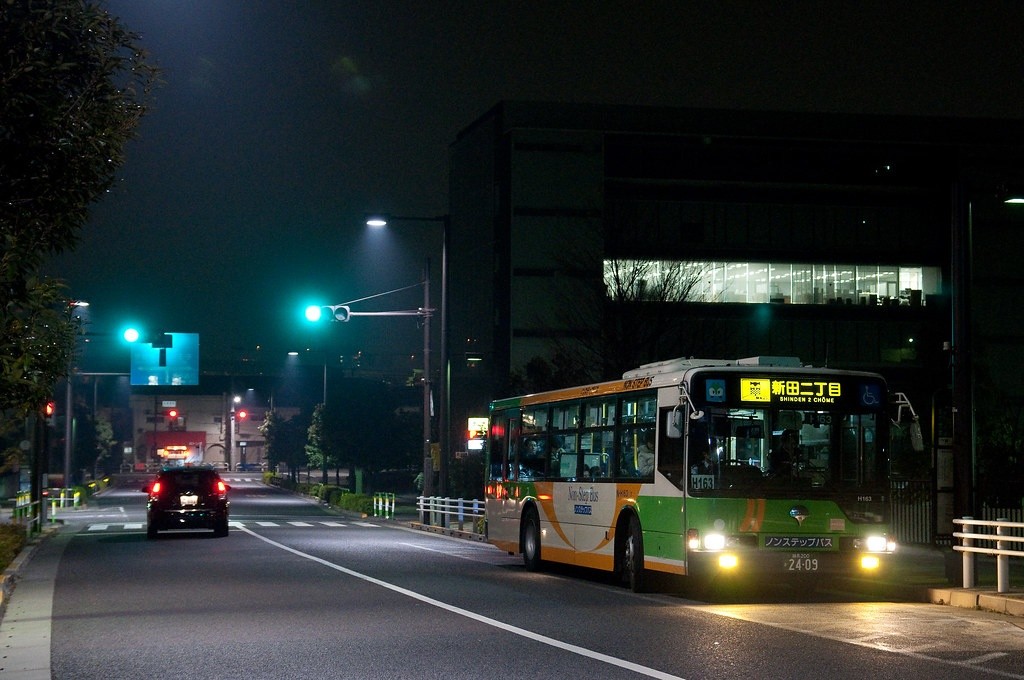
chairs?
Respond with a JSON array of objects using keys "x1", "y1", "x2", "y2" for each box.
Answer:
[{"x1": 625, "y1": 452, "x2": 640, "y2": 477}]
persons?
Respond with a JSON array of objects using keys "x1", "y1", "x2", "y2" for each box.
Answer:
[
  {"x1": 681, "y1": 437, "x2": 738, "y2": 491},
  {"x1": 769, "y1": 431, "x2": 800, "y2": 496},
  {"x1": 498, "y1": 419, "x2": 656, "y2": 479}
]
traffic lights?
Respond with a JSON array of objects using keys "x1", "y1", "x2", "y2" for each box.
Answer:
[
  {"x1": 123, "y1": 328, "x2": 163, "y2": 343},
  {"x1": 169, "y1": 410, "x2": 176, "y2": 417},
  {"x1": 43, "y1": 401, "x2": 55, "y2": 418},
  {"x1": 240, "y1": 412, "x2": 247, "y2": 418},
  {"x1": 304, "y1": 305, "x2": 350, "y2": 323}
]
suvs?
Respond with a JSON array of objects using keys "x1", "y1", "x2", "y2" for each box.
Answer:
[{"x1": 141, "y1": 464, "x2": 232, "y2": 538}]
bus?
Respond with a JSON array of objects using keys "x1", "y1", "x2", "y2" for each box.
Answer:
[
  {"x1": 164, "y1": 446, "x2": 187, "y2": 470},
  {"x1": 485, "y1": 357, "x2": 924, "y2": 604}
]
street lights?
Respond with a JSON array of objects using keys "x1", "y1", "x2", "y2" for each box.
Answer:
[
  {"x1": 288, "y1": 352, "x2": 330, "y2": 503},
  {"x1": 66, "y1": 300, "x2": 89, "y2": 506},
  {"x1": 366, "y1": 214, "x2": 450, "y2": 527},
  {"x1": 229, "y1": 387, "x2": 255, "y2": 472}
]
